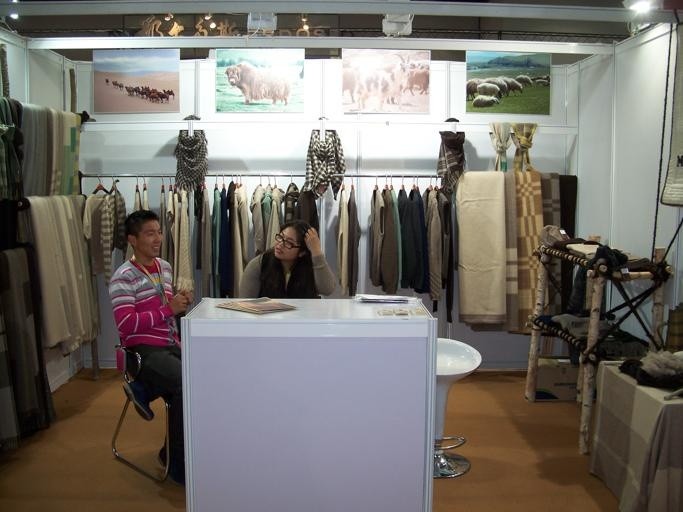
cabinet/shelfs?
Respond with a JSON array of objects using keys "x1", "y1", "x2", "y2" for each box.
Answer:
[
  {"x1": 527, "y1": 229, "x2": 661, "y2": 446},
  {"x1": 181, "y1": 297, "x2": 438, "y2": 512}
]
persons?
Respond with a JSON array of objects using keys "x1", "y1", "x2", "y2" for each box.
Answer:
[
  {"x1": 109, "y1": 209, "x2": 197, "y2": 486},
  {"x1": 237, "y1": 219, "x2": 337, "y2": 297}
]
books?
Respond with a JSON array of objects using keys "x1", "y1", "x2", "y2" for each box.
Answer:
[{"x1": 213, "y1": 297, "x2": 296, "y2": 316}]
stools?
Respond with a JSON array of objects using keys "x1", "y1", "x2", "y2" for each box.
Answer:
[{"x1": 433, "y1": 336, "x2": 483, "y2": 479}]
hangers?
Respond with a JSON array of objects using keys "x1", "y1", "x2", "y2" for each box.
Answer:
[{"x1": 93, "y1": 173, "x2": 442, "y2": 199}]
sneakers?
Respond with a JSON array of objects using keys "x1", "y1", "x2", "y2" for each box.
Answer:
[
  {"x1": 157, "y1": 443, "x2": 185, "y2": 489},
  {"x1": 120, "y1": 379, "x2": 155, "y2": 421}
]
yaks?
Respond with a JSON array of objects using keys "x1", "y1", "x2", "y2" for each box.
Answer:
[{"x1": 225, "y1": 60, "x2": 291, "y2": 104}]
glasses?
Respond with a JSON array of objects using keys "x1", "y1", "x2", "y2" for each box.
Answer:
[{"x1": 273, "y1": 233, "x2": 303, "y2": 250}]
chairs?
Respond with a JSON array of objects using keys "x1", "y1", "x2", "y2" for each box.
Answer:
[{"x1": 110, "y1": 343, "x2": 172, "y2": 483}]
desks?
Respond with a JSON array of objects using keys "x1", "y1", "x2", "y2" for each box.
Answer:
[{"x1": 595, "y1": 353, "x2": 682, "y2": 511}]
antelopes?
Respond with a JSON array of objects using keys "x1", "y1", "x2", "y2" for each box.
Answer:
[{"x1": 343, "y1": 52, "x2": 430, "y2": 109}]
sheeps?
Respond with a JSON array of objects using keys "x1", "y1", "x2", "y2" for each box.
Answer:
[
  {"x1": 471, "y1": 94, "x2": 500, "y2": 108},
  {"x1": 466, "y1": 74, "x2": 550, "y2": 95}
]
